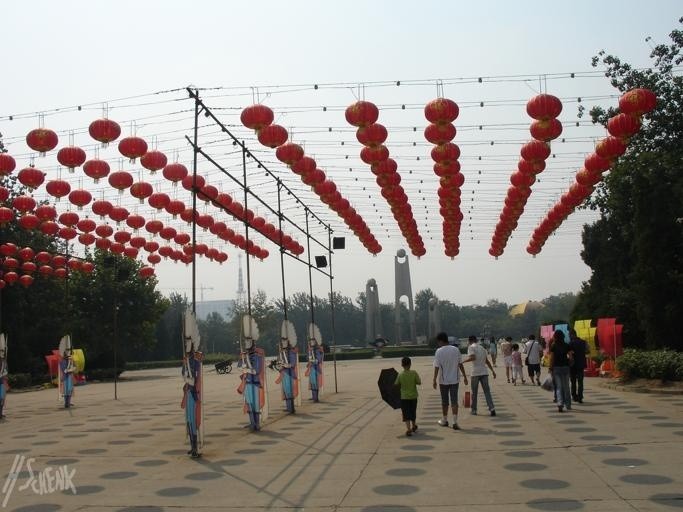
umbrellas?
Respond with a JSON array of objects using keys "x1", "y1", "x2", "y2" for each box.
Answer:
[
  {"x1": 508, "y1": 300, "x2": 548, "y2": 320},
  {"x1": 377, "y1": 367, "x2": 402, "y2": 409}
]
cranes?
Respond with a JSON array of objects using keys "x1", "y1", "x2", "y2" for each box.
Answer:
[{"x1": 156, "y1": 282, "x2": 213, "y2": 301}]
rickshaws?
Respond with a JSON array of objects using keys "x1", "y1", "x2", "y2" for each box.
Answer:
[
  {"x1": 206, "y1": 358, "x2": 232, "y2": 375},
  {"x1": 265, "y1": 359, "x2": 279, "y2": 371}
]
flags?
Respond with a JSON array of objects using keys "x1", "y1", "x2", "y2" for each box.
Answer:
[
  {"x1": 1, "y1": 86, "x2": 658, "y2": 287},
  {"x1": 540, "y1": 318, "x2": 623, "y2": 367}
]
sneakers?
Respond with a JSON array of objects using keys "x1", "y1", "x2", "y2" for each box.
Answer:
[{"x1": 405, "y1": 379, "x2": 582, "y2": 437}]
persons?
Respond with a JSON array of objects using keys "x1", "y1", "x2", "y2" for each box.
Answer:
[
  {"x1": 236, "y1": 313, "x2": 264, "y2": 431},
  {"x1": 461, "y1": 335, "x2": 498, "y2": 416},
  {"x1": 305, "y1": 322, "x2": 325, "y2": 403},
  {"x1": 480, "y1": 327, "x2": 591, "y2": 413},
  {"x1": 179, "y1": 305, "x2": 204, "y2": 459},
  {"x1": 0, "y1": 332, "x2": 9, "y2": 423},
  {"x1": 270, "y1": 320, "x2": 300, "y2": 413},
  {"x1": 59, "y1": 334, "x2": 76, "y2": 409},
  {"x1": 432, "y1": 331, "x2": 469, "y2": 429},
  {"x1": 598, "y1": 356, "x2": 615, "y2": 377},
  {"x1": 392, "y1": 356, "x2": 422, "y2": 437}
]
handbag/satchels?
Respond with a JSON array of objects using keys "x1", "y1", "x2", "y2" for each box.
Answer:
[{"x1": 525, "y1": 357, "x2": 528, "y2": 365}]
